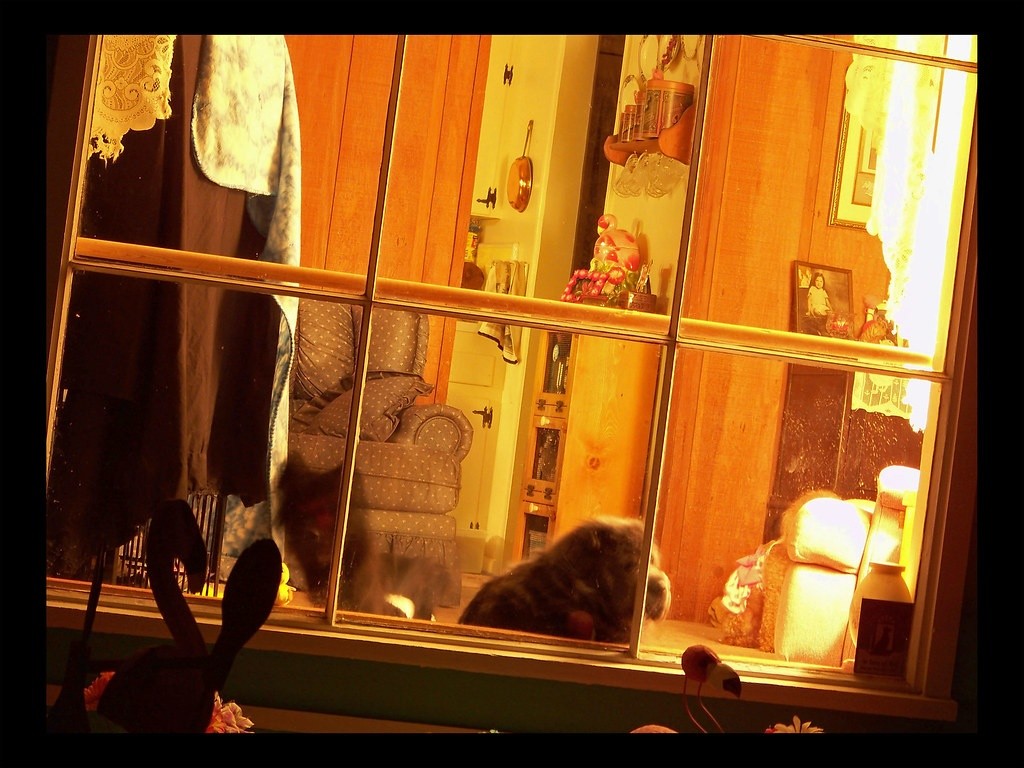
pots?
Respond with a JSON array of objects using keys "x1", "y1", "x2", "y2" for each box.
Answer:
[{"x1": 507, "y1": 120, "x2": 535, "y2": 213}]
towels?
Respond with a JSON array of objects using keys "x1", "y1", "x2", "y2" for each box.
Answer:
[
  {"x1": 189, "y1": 34, "x2": 303, "y2": 572},
  {"x1": 477, "y1": 260, "x2": 526, "y2": 365}
]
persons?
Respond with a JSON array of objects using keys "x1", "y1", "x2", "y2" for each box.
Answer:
[{"x1": 805, "y1": 272, "x2": 834, "y2": 318}]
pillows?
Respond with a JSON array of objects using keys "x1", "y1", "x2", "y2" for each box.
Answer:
[{"x1": 289, "y1": 370, "x2": 434, "y2": 443}]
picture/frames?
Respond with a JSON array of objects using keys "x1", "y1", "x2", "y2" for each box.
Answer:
[
  {"x1": 826, "y1": 108, "x2": 879, "y2": 231},
  {"x1": 792, "y1": 260, "x2": 855, "y2": 340}
]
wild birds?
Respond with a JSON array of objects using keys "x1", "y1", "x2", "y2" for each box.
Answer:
[
  {"x1": 627, "y1": 646, "x2": 743, "y2": 733},
  {"x1": 45, "y1": 497, "x2": 284, "y2": 733}
]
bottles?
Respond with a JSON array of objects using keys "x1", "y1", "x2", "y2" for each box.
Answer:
[
  {"x1": 463, "y1": 224, "x2": 480, "y2": 263},
  {"x1": 549, "y1": 334, "x2": 565, "y2": 394}
]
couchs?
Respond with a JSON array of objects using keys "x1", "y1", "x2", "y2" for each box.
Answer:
[{"x1": 287, "y1": 300, "x2": 473, "y2": 606}]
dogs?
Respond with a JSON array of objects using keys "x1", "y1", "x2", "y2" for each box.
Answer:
[
  {"x1": 277, "y1": 451, "x2": 408, "y2": 621},
  {"x1": 454, "y1": 514, "x2": 672, "y2": 649}
]
